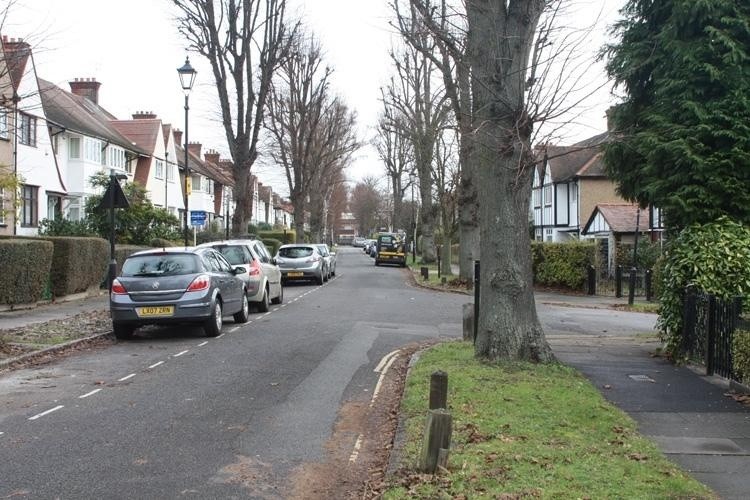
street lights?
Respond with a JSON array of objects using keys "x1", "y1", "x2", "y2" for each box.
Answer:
[
  {"x1": 177, "y1": 56, "x2": 198, "y2": 245},
  {"x1": 409, "y1": 169, "x2": 417, "y2": 263}
]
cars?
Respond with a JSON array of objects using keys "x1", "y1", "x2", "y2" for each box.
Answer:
[
  {"x1": 272, "y1": 244, "x2": 336, "y2": 284},
  {"x1": 196, "y1": 238, "x2": 283, "y2": 312},
  {"x1": 110, "y1": 245, "x2": 249, "y2": 339},
  {"x1": 351, "y1": 233, "x2": 408, "y2": 268}
]
